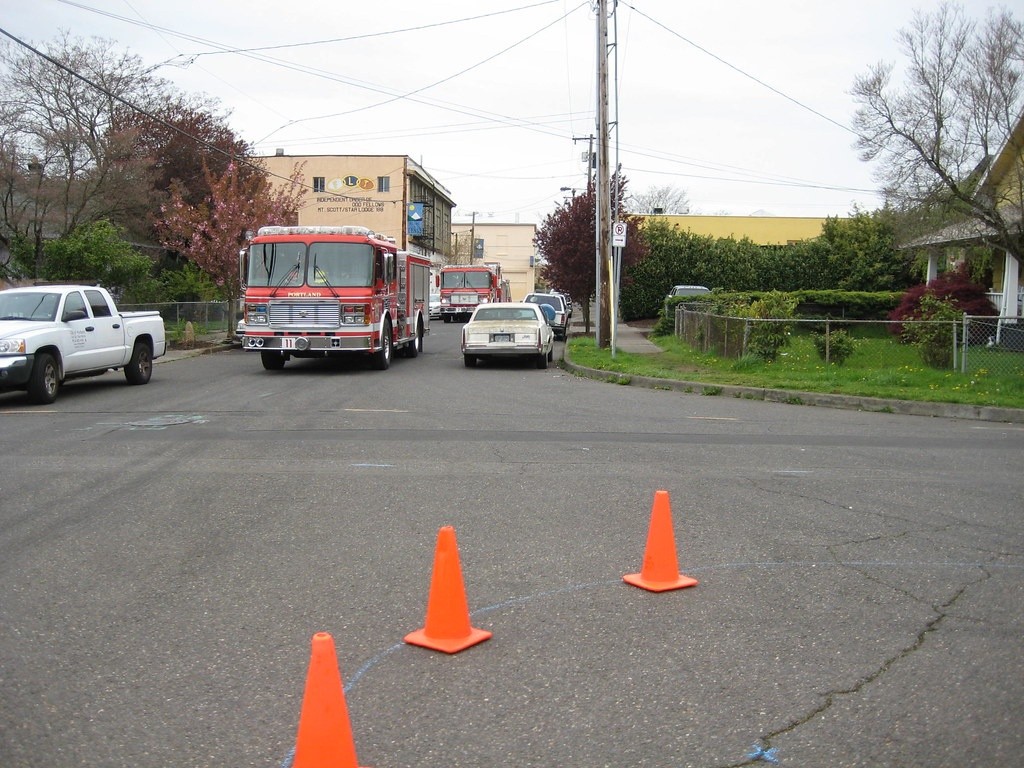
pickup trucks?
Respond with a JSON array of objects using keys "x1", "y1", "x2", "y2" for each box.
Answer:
[{"x1": 0, "y1": 284, "x2": 168, "y2": 405}]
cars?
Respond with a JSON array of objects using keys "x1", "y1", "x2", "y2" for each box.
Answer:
[
  {"x1": 665, "y1": 284, "x2": 710, "y2": 318},
  {"x1": 519, "y1": 290, "x2": 573, "y2": 338},
  {"x1": 429, "y1": 295, "x2": 443, "y2": 320},
  {"x1": 460, "y1": 301, "x2": 556, "y2": 369}
]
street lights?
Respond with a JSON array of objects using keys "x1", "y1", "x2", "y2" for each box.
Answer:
[
  {"x1": 560, "y1": 187, "x2": 576, "y2": 216},
  {"x1": 451, "y1": 231, "x2": 457, "y2": 265}
]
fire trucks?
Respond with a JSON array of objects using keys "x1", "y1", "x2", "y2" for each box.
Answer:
[
  {"x1": 435, "y1": 263, "x2": 512, "y2": 324},
  {"x1": 234, "y1": 224, "x2": 434, "y2": 371}
]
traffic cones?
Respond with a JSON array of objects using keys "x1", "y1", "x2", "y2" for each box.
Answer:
[
  {"x1": 620, "y1": 488, "x2": 699, "y2": 594},
  {"x1": 402, "y1": 526, "x2": 494, "y2": 655},
  {"x1": 287, "y1": 632, "x2": 370, "y2": 768}
]
